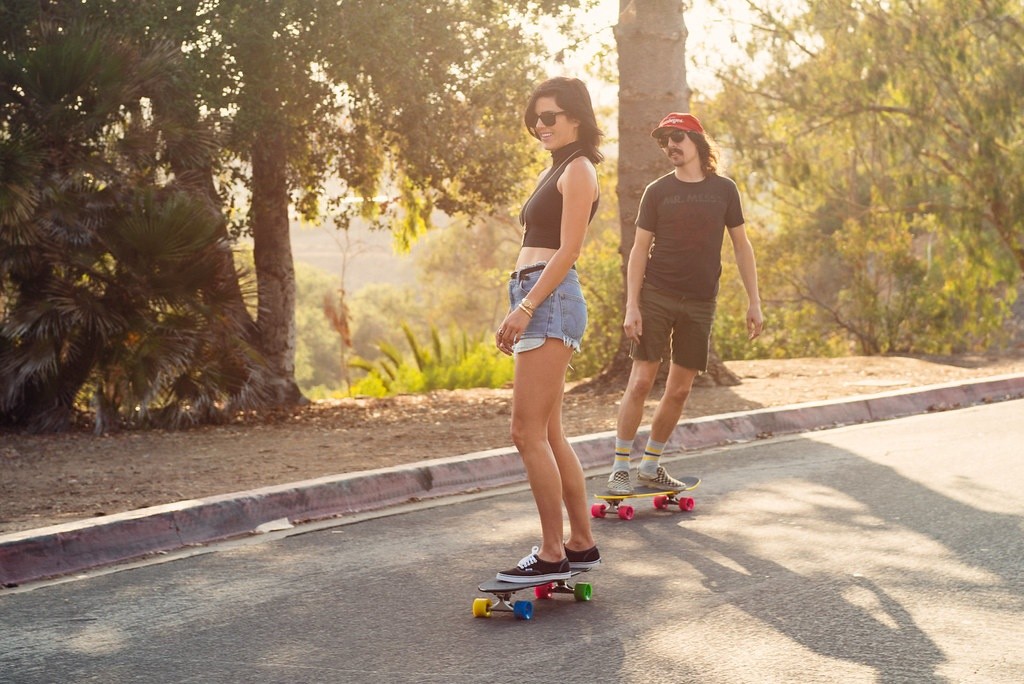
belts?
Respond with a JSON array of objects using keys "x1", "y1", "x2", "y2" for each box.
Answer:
[{"x1": 511, "y1": 263, "x2": 576, "y2": 281}]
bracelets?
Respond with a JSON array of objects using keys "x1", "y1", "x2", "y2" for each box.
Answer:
[{"x1": 519, "y1": 303, "x2": 532, "y2": 318}]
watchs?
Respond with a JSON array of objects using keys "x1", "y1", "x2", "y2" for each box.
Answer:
[{"x1": 522, "y1": 298, "x2": 536, "y2": 312}]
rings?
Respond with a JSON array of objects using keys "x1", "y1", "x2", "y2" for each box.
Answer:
[{"x1": 498, "y1": 330, "x2": 504, "y2": 335}]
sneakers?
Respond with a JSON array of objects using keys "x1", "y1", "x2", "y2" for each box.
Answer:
[
  {"x1": 636, "y1": 466, "x2": 686, "y2": 491},
  {"x1": 496, "y1": 546, "x2": 572, "y2": 584},
  {"x1": 606, "y1": 469, "x2": 634, "y2": 495},
  {"x1": 563, "y1": 541, "x2": 601, "y2": 568}
]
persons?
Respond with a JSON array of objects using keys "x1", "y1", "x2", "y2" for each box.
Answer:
[
  {"x1": 494, "y1": 76, "x2": 603, "y2": 583},
  {"x1": 610, "y1": 112, "x2": 764, "y2": 497}
]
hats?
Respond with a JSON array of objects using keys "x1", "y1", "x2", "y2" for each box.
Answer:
[{"x1": 651, "y1": 113, "x2": 703, "y2": 138}]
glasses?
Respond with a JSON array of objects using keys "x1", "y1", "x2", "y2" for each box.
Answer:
[
  {"x1": 657, "y1": 130, "x2": 690, "y2": 148},
  {"x1": 531, "y1": 111, "x2": 565, "y2": 128}
]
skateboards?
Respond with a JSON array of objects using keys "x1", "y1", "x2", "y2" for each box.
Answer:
[
  {"x1": 591, "y1": 476, "x2": 701, "y2": 520},
  {"x1": 473, "y1": 567, "x2": 592, "y2": 620}
]
